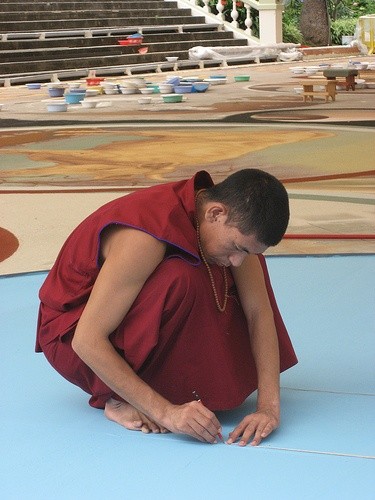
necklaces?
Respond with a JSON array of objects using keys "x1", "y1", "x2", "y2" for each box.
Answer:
[{"x1": 194, "y1": 189, "x2": 229, "y2": 313}]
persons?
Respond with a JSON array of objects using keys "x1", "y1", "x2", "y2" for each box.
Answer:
[{"x1": 35, "y1": 168, "x2": 299, "y2": 447}]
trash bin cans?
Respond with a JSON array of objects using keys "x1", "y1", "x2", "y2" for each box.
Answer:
[{"x1": 359, "y1": 14, "x2": 375, "y2": 55}]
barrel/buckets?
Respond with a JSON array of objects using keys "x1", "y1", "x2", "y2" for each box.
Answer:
[{"x1": 342, "y1": 36, "x2": 354, "y2": 45}]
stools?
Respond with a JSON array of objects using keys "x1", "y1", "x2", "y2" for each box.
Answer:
[
  {"x1": 299, "y1": 78, "x2": 338, "y2": 102},
  {"x1": 323, "y1": 69, "x2": 358, "y2": 92}
]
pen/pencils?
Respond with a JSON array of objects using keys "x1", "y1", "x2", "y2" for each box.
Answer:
[{"x1": 192, "y1": 391, "x2": 225, "y2": 444}]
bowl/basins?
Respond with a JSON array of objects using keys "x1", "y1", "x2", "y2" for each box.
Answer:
[
  {"x1": 288, "y1": 60, "x2": 375, "y2": 74},
  {"x1": 294, "y1": 78, "x2": 375, "y2": 93},
  {"x1": 138, "y1": 47, "x2": 148, "y2": 54},
  {"x1": 25, "y1": 75, "x2": 251, "y2": 112},
  {"x1": 118, "y1": 37, "x2": 144, "y2": 46},
  {"x1": 165, "y1": 56, "x2": 179, "y2": 62}
]
